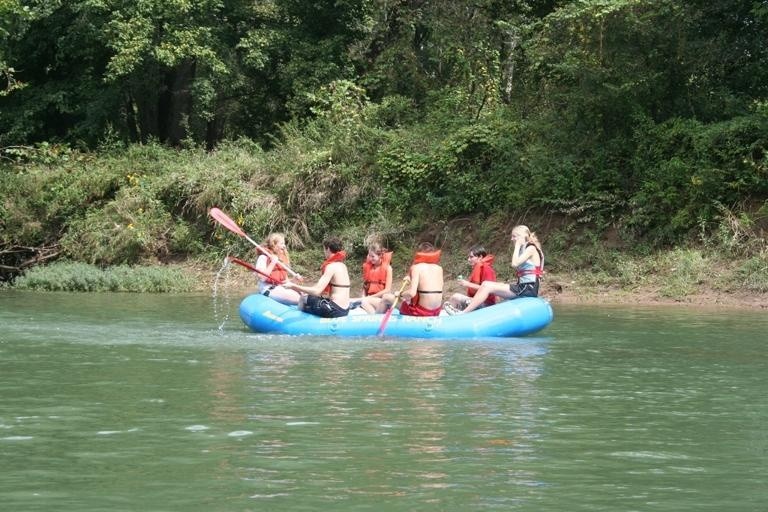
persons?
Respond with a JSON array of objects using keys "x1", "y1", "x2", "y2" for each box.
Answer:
[
  {"x1": 374, "y1": 241, "x2": 444, "y2": 316},
  {"x1": 255, "y1": 232, "x2": 307, "y2": 305},
  {"x1": 449, "y1": 245, "x2": 500, "y2": 311},
  {"x1": 280, "y1": 238, "x2": 351, "y2": 318},
  {"x1": 443, "y1": 225, "x2": 544, "y2": 316},
  {"x1": 349, "y1": 242, "x2": 393, "y2": 315}
]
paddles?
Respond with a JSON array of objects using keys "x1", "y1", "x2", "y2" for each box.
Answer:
[
  {"x1": 376, "y1": 281, "x2": 408, "y2": 336},
  {"x1": 210, "y1": 207, "x2": 305, "y2": 282},
  {"x1": 231, "y1": 257, "x2": 302, "y2": 297}
]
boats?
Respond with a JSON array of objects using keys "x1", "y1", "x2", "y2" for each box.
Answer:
[{"x1": 239, "y1": 290, "x2": 554, "y2": 341}]
